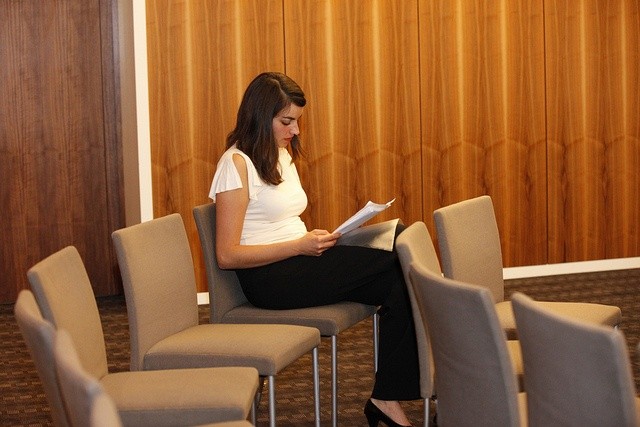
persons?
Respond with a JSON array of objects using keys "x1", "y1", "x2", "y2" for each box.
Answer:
[{"x1": 208, "y1": 71, "x2": 439, "y2": 427}]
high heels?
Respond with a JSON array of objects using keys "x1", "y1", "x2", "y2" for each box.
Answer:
[{"x1": 363, "y1": 401, "x2": 413, "y2": 427}]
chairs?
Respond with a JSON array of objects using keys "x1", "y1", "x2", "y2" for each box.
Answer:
[
  {"x1": 26, "y1": 246, "x2": 260, "y2": 427},
  {"x1": 396, "y1": 220, "x2": 523, "y2": 427},
  {"x1": 193, "y1": 204, "x2": 380, "y2": 427},
  {"x1": 410, "y1": 262, "x2": 529, "y2": 427},
  {"x1": 111, "y1": 213, "x2": 321, "y2": 427},
  {"x1": 433, "y1": 196, "x2": 623, "y2": 331},
  {"x1": 511, "y1": 292, "x2": 640, "y2": 427},
  {"x1": 14, "y1": 290, "x2": 254, "y2": 427}
]
